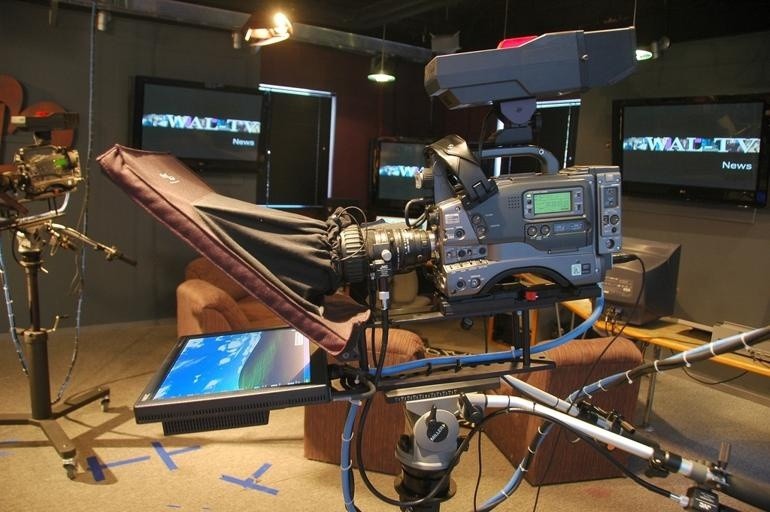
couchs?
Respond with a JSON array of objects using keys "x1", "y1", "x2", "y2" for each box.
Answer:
[
  {"x1": 302, "y1": 326, "x2": 433, "y2": 476},
  {"x1": 176, "y1": 256, "x2": 292, "y2": 336},
  {"x1": 482, "y1": 333, "x2": 643, "y2": 488}
]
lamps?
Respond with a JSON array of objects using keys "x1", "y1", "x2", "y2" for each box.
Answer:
[
  {"x1": 635, "y1": 35, "x2": 670, "y2": 62},
  {"x1": 97, "y1": 10, "x2": 108, "y2": 31},
  {"x1": 367, "y1": 24, "x2": 397, "y2": 83},
  {"x1": 244, "y1": 8, "x2": 294, "y2": 48}
]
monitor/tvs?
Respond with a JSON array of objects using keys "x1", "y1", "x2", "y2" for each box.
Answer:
[
  {"x1": 611, "y1": 92, "x2": 770, "y2": 209},
  {"x1": 130, "y1": 75, "x2": 269, "y2": 175},
  {"x1": 369, "y1": 137, "x2": 441, "y2": 208},
  {"x1": 134, "y1": 325, "x2": 328, "y2": 439}
]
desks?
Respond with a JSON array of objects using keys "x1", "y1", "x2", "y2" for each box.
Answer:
[{"x1": 587, "y1": 317, "x2": 770, "y2": 433}]
